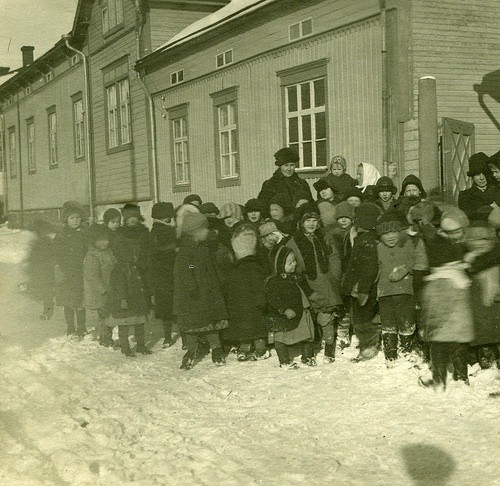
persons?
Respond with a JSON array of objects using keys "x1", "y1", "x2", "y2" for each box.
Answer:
[{"x1": 24, "y1": 148, "x2": 500, "y2": 391}]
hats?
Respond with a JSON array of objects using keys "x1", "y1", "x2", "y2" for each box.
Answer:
[
  {"x1": 398, "y1": 198, "x2": 417, "y2": 216},
  {"x1": 467, "y1": 152, "x2": 492, "y2": 177},
  {"x1": 334, "y1": 201, "x2": 354, "y2": 220},
  {"x1": 273, "y1": 148, "x2": 299, "y2": 166},
  {"x1": 216, "y1": 202, "x2": 244, "y2": 222},
  {"x1": 59, "y1": 194, "x2": 220, "y2": 244},
  {"x1": 270, "y1": 193, "x2": 292, "y2": 213},
  {"x1": 377, "y1": 214, "x2": 400, "y2": 235},
  {"x1": 401, "y1": 174, "x2": 424, "y2": 195},
  {"x1": 342, "y1": 185, "x2": 362, "y2": 201},
  {"x1": 354, "y1": 201, "x2": 380, "y2": 229},
  {"x1": 313, "y1": 177, "x2": 339, "y2": 199},
  {"x1": 269, "y1": 244, "x2": 294, "y2": 274},
  {"x1": 259, "y1": 218, "x2": 291, "y2": 244},
  {"x1": 244, "y1": 199, "x2": 265, "y2": 213},
  {"x1": 328, "y1": 156, "x2": 347, "y2": 172},
  {"x1": 299, "y1": 203, "x2": 320, "y2": 224},
  {"x1": 373, "y1": 176, "x2": 397, "y2": 195}
]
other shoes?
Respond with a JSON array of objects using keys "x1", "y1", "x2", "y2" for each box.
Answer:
[{"x1": 38, "y1": 305, "x2": 499, "y2": 392}]
